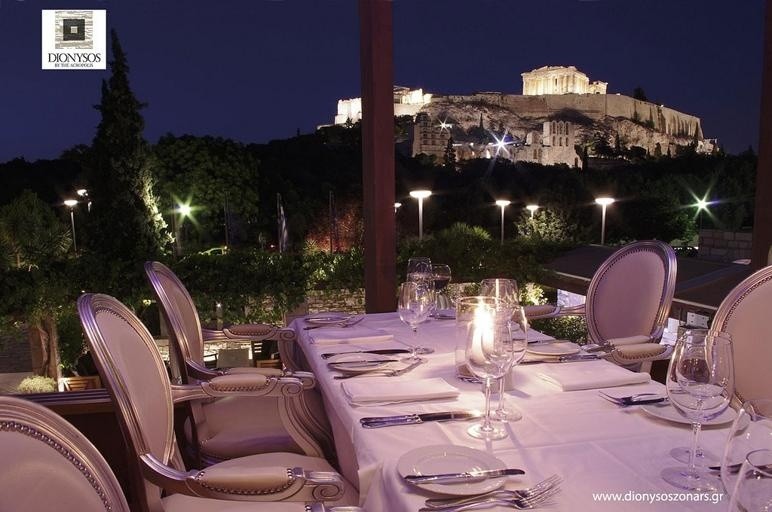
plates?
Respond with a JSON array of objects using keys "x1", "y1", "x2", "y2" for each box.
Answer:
[
  {"x1": 525, "y1": 340, "x2": 581, "y2": 354},
  {"x1": 639, "y1": 394, "x2": 737, "y2": 425},
  {"x1": 328, "y1": 353, "x2": 389, "y2": 372},
  {"x1": 306, "y1": 312, "x2": 350, "y2": 324},
  {"x1": 399, "y1": 444, "x2": 508, "y2": 495}
]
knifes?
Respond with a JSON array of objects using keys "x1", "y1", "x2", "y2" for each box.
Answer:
[
  {"x1": 708, "y1": 459, "x2": 772, "y2": 478},
  {"x1": 405, "y1": 469, "x2": 525, "y2": 482},
  {"x1": 364, "y1": 416, "x2": 471, "y2": 429},
  {"x1": 321, "y1": 349, "x2": 410, "y2": 359}
]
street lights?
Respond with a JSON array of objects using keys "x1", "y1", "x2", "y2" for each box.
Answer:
[
  {"x1": 526, "y1": 202, "x2": 540, "y2": 223},
  {"x1": 592, "y1": 195, "x2": 613, "y2": 240},
  {"x1": 64, "y1": 185, "x2": 91, "y2": 253},
  {"x1": 408, "y1": 185, "x2": 434, "y2": 240},
  {"x1": 492, "y1": 197, "x2": 512, "y2": 243}
]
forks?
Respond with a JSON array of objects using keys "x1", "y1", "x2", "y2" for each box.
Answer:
[
  {"x1": 418, "y1": 486, "x2": 562, "y2": 511},
  {"x1": 596, "y1": 387, "x2": 667, "y2": 409},
  {"x1": 303, "y1": 319, "x2": 367, "y2": 329},
  {"x1": 360, "y1": 410, "x2": 471, "y2": 423},
  {"x1": 344, "y1": 360, "x2": 421, "y2": 377},
  {"x1": 427, "y1": 474, "x2": 563, "y2": 507},
  {"x1": 333, "y1": 369, "x2": 408, "y2": 379}
]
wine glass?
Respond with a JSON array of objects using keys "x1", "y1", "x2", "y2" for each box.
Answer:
[{"x1": 399, "y1": 255, "x2": 772, "y2": 512}]
